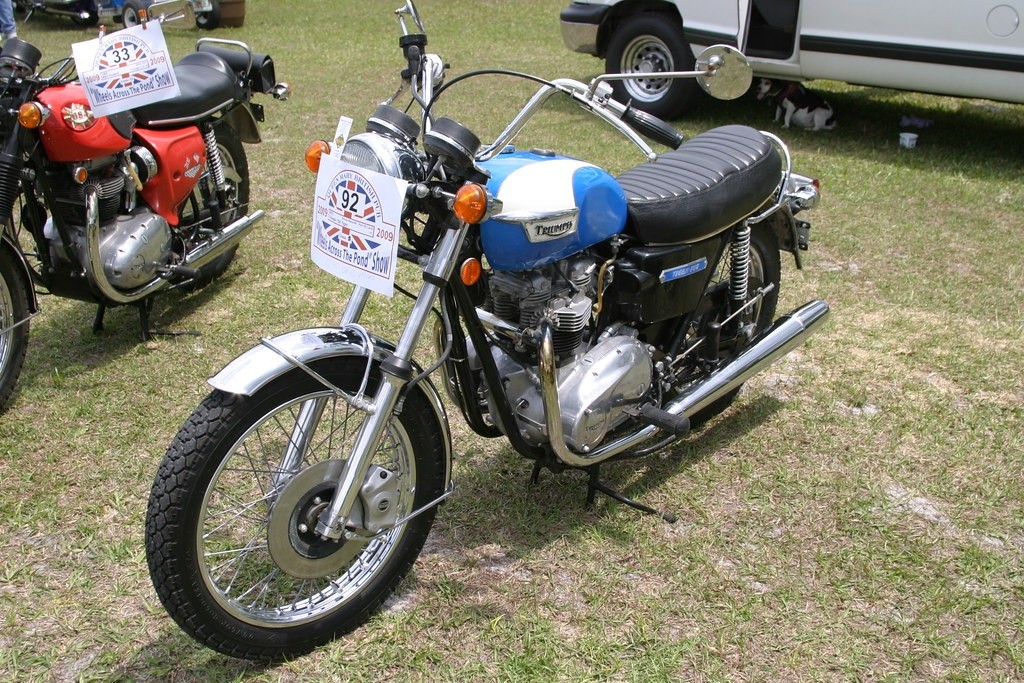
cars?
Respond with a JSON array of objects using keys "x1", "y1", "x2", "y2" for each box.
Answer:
[{"x1": 557, "y1": 1, "x2": 1024, "y2": 123}]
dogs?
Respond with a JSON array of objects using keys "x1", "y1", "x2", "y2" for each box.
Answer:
[{"x1": 756, "y1": 77, "x2": 838, "y2": 132}]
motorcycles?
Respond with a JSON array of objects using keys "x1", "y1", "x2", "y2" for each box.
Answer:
[
  {"x1": 0, "y1": 0, "x2": 294, "y2": 416},
  {"x1": 145, "y1": 0, "x2": 832, "y2": 663}
]
